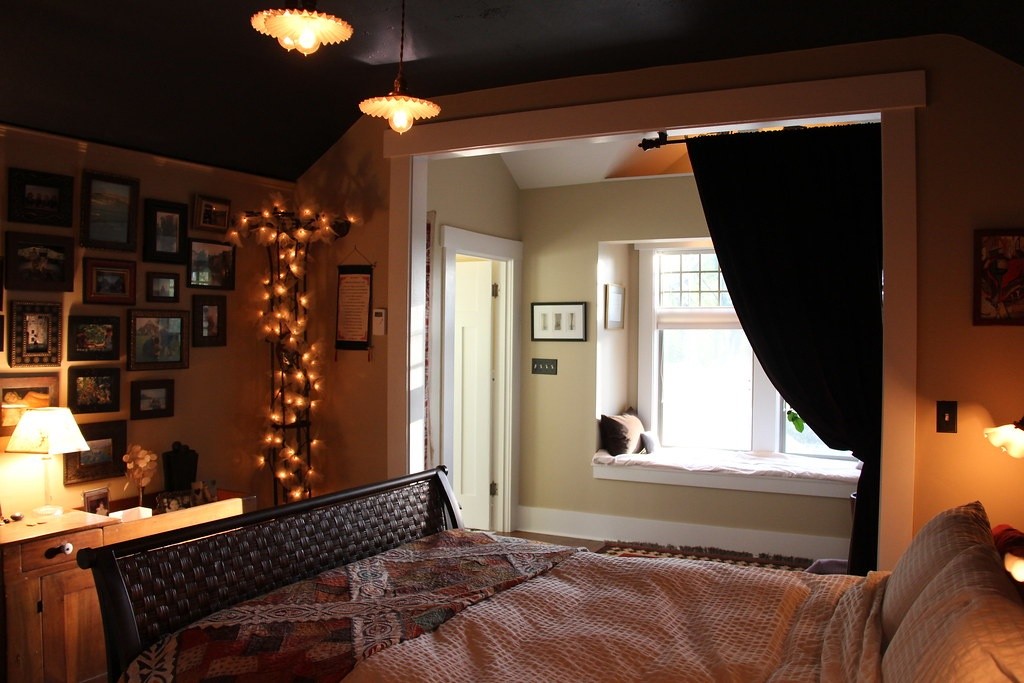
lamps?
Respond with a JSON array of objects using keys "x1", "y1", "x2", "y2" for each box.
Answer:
[
  {"x1": 4, "y1": 406, "x2": 90, "y2": 519},
  {"x1": 358, "y1": 0, "x2": 441, "y2": 137},
  {"x1": 982, "y1": 415, "x2": 1024, "y2": 460},
  {"x1": 249, "y1": 0, "x2": 355, "y2": 58}
]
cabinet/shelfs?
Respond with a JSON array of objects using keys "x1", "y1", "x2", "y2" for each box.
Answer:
[{"x1": 0, "y1": 487, "x2": 258, "y2": 683}]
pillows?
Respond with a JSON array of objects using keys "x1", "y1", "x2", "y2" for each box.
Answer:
[
  {"x1": 883, "y1": 544, "x2": 1024, "y2": 683},
  {"x1": 600, "y1": 406, "x2": 646, "y2": 457},
  {"x1": 641, "y1": 430, "x2": 661, "y2": 454},
  {"x1": 880, "y1": 501, "x2": 1000, "y2": 652}
]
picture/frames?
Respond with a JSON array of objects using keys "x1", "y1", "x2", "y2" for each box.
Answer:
[
  {"x1": 531, "y1": 302, "x2": 587, "y2": 342},
  {"x1": 971, "y1": 228, "x2": 1024, "y2": 325},
  {"x1": 0, "y1": 166, "x2": 239, "y2": 486},
  {"x1": 156, "y1": 489, "x2": 194, "y2": 514},
  {"x1": 605, "y1": 283, "x2": 626, "y2": 330},
  {"x1": 81, "y1": 484, "x2": 110, "y2": 517}
]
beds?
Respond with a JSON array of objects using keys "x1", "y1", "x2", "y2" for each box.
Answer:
[{"x1": 75, "y1": 464, "x2": 1024, "y2": 683}]
